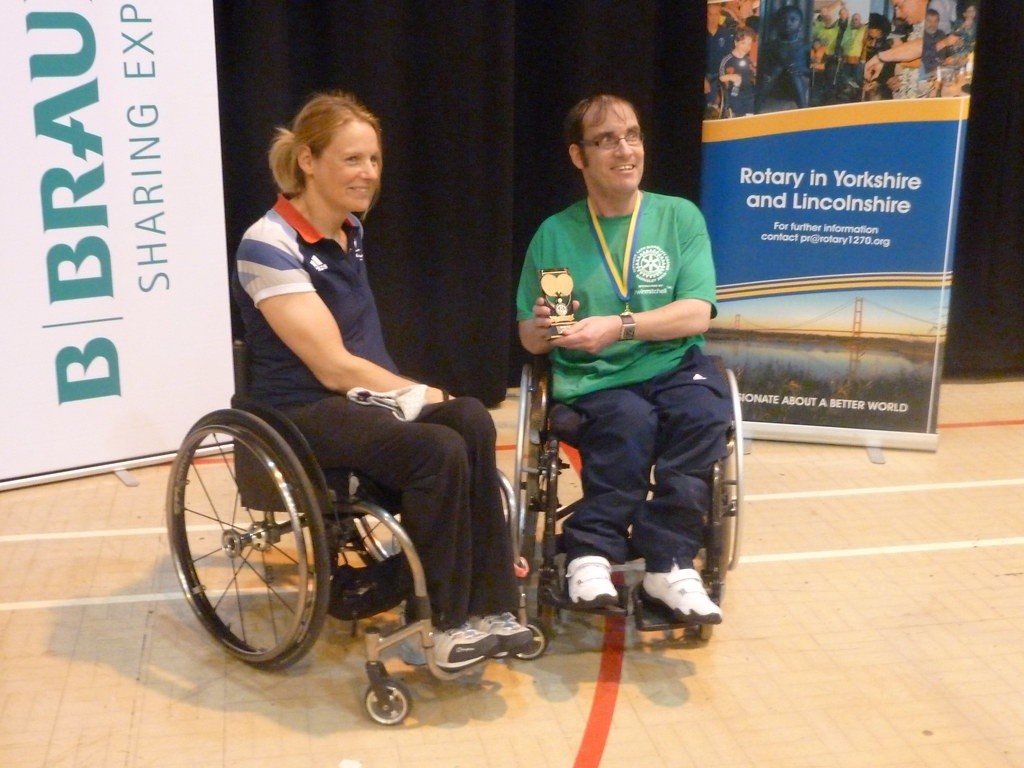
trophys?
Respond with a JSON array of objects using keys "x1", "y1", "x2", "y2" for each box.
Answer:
[{"x1": 540, "y1": 268, "x2": 578, "y2": 342}]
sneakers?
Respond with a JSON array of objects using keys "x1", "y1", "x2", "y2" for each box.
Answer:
[
  {"x1": 470, "y1": 612, "x2": 533, "y2": 653},
  {"x1": 399, "y1": 621, "x2": 499, "y2": 671}
]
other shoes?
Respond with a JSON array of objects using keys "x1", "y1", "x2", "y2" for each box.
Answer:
[
  {"x1": 639, "y1": 562, "x2": 722, "y2": 625},
  {"x1": 565, "y1": 555, "x2": 619, "y2": 611}
]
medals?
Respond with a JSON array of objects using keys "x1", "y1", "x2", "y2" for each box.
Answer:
[{"x1": 621, "y1": 311, "x2": 633, "y2": 316}]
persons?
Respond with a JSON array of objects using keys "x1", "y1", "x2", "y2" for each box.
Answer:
[
  {"x1": 233, "y1": 92, "x2": 537, "y2": 676},
  {"x1": 703, "y1": 0, "x2": 977, "y2": 118},
  {"x1": 515, "y1": 95, "x2": 734, "y2": 625}
]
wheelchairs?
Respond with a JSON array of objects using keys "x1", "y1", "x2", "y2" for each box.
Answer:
[
  {"x1": 509, "y1": 360, "x2": 744, "y2": 642},
  {"x1": 165, "y1": 340, "x2": 549, "y2": 727}
]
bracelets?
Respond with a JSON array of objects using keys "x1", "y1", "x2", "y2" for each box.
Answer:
[
  {"x1": 877, "y1": 52, "x2": 886, "y2": 64},
  {"x1": 442, "y1": 390, "x2": 449, "y2": 402}
]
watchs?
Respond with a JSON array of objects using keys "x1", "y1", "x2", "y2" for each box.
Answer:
[{"x1": 618, "y1": 314, "x2": 636, "y2": 341}]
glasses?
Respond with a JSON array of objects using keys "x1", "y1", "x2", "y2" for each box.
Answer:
[{"x1": 580, "y1": 131, "x2": 644, "y2": 149}]
